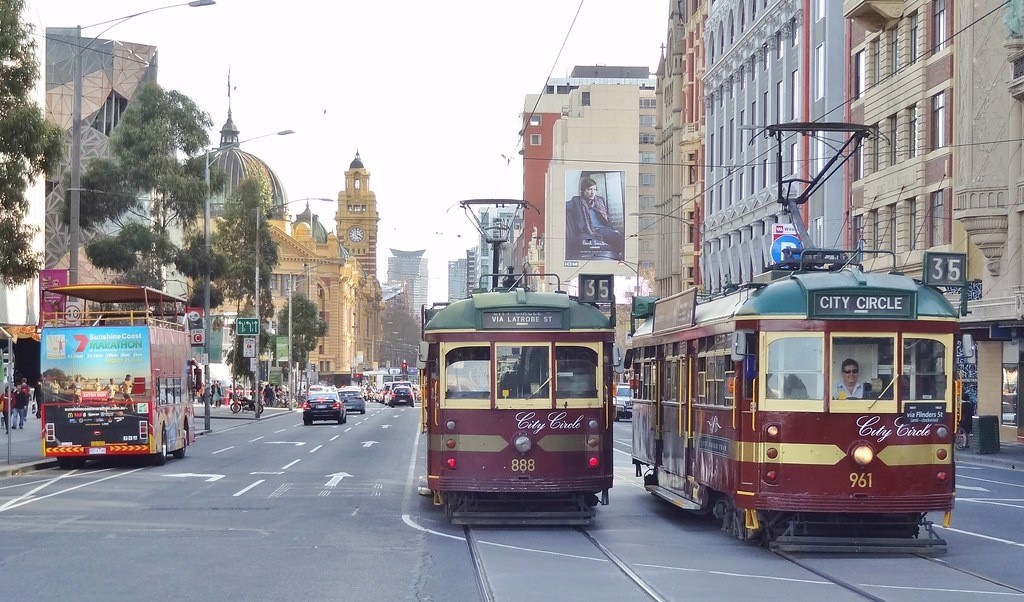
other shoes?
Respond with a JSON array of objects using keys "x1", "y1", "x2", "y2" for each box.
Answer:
[
  {"x1": 6, "y1": 431, "x2": 8, "y2": 434},
  {"x1": 20, "y1": 426, "x2": 23, "y2": 429},
  {"x1": 11, "y1": 425, "x2": 16, "y2": 429}
]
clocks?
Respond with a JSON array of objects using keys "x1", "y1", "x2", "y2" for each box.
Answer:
[{"x1": 347, "y1": 226, "x2": 366, "y2": 244}]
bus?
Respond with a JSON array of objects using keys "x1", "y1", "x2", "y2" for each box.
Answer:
[
  {"x1": 34, "y1": 283, "x2": 195, "y2": 467},
  {"x1": 622, "y1": 123, "x2": 977, "y2": 555},
  {"x1": 416, "y1": 197, "x2": 618, "y2": 528}
]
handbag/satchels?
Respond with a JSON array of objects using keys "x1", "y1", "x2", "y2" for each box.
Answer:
[
  {"x1": 31, "y1": 404, "x2": 36, "y2": 414},
  {"x1": 0, "y1": 393, "x2": 5, "y2": 411}
]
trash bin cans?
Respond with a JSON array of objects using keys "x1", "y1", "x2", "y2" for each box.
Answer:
[
  {"x1": 972, "y1": 416, "x2": 1001, "y2": 454},
  {"x1": 198, "y1": 397, "x2": 202, "y2": 403}
]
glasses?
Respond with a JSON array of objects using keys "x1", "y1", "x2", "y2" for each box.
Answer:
[{"x1": 843, "y1": 368, "x2": 858, "y2": 374}]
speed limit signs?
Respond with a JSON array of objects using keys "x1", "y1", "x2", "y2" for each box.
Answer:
[{"x1": 64, "y1": 302, "x2": 82, "y2": 327}]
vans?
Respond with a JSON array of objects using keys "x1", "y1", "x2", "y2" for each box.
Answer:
[
  {"x1": 1002, "y1": 402, "x2": 1018, "y2": 422},
  {"x1": 615, "y1": 384, "x2": 636, "y2": 420}
]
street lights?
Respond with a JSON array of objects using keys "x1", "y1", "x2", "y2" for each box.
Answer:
[
  {"x1": 202, "y1": 131, "x2": 296, "y2": 433},
  {"x1": 355, "y1": 373, "x2": 362, "y2": 379},
  {"x1": 287, "y1": 262, "x2": 342, "y2": 410},
  {"x1": 68, "y1": 0, "x2": 215, "y2": 327},
  {"x1": 255, "y1": 198, "x2": 335, "y2": 419},
  {"x1": 401, "y1": 363, "x2": 407, "y2": 373}
]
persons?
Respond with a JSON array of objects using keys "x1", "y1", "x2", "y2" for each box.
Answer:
[
  {"x1": 263, "y1": 383, "x2": 283, "y2": 407},
  {"x1": 958, "y1": 393, "x2": 973, "y2": 448},
  {"x1": 832, "y1": 359, "x2": 863, "y2": 400},
  {"x1": 565, "y1": 178, "x2": 625, "y2": 261},
  {"x1": 0, "y1": 378, "x2": 41, "y2": 434},
  {"x1": 50, "y1": 374, "x2": 136, "y2": 413},
  {"x1": 193, "y1": 380, "x2": 223, "y2": 408}
]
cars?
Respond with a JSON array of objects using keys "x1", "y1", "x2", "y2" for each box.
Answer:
[{"x1": 302, "y1": 382, "x2": 415, "y2": 425}]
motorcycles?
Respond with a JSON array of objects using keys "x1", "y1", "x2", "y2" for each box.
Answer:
[{"x1": 230, "y1": 395, "x2": 264, "y2": 413}]
